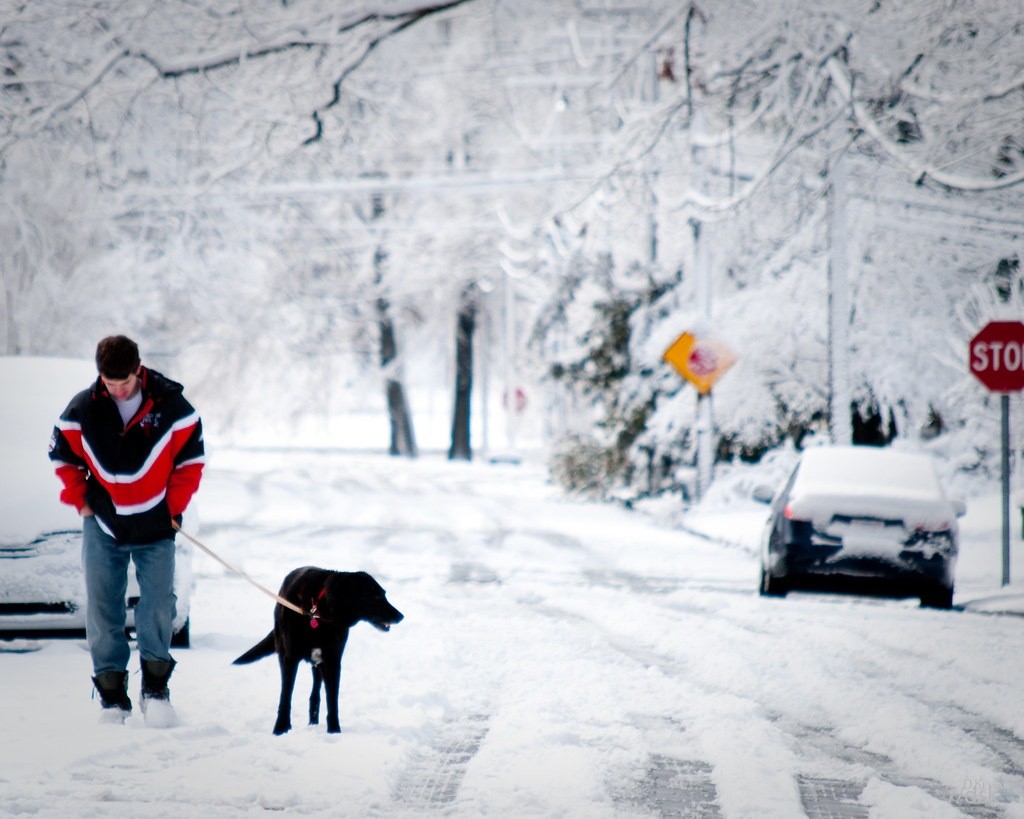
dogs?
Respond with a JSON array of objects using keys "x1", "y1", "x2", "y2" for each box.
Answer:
[{"x1": 233, "y1": 566, "x2": 404, "y2": 736}]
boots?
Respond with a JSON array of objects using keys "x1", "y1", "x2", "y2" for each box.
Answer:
[
  {"x1": 89, "y1": 669, "x2": 132, "y2": 726},
  {"x1": 138, "y1": 656, "x2": 178, "y2": 729}
]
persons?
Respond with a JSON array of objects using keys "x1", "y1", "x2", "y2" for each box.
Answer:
[{"x1": 47, "y1": 333, "x2": 204, "y2": 727}]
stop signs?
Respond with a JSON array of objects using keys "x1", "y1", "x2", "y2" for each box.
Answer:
[{"x1": 968, "y1": 319, "x2": 1023, "y2": 394}]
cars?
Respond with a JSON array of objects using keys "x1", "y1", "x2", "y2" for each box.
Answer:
[
  {"x1": 0, "y1": 352, "x2": 188, "y2": 648},
  {"x1": 761, "y1": 444, "x2": 971, "y2": 612}
]
